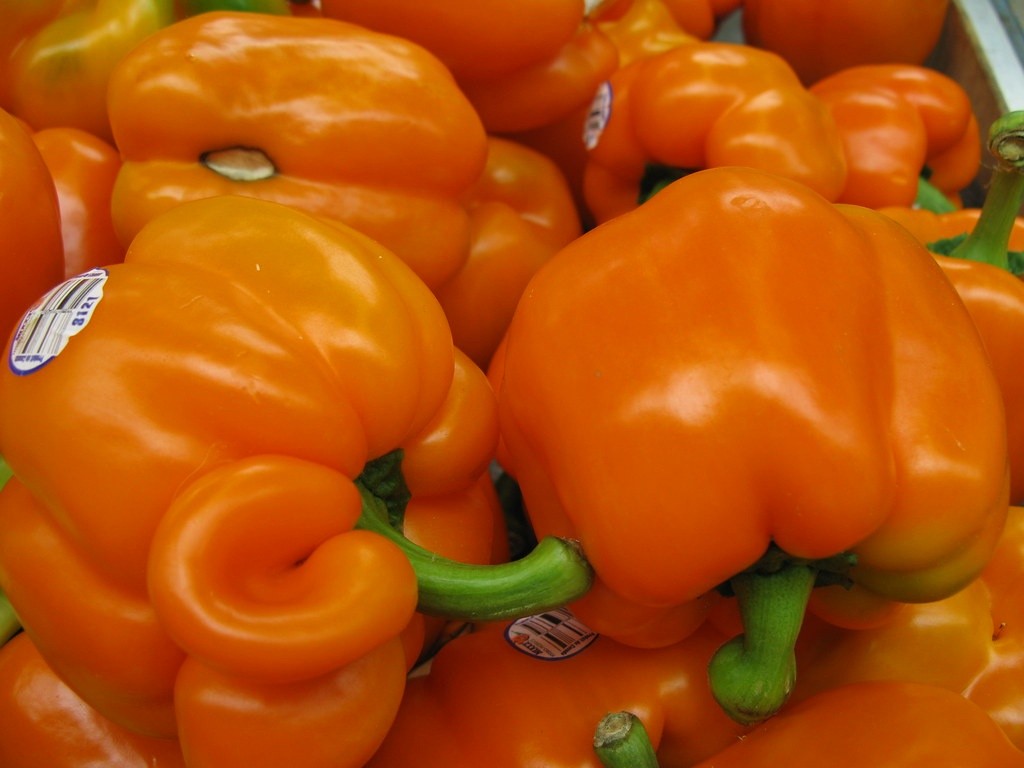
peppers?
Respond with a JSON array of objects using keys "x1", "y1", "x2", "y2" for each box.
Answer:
[{"x1": 0, "y1": 0, "x2": 1024, "y2": 768}]
